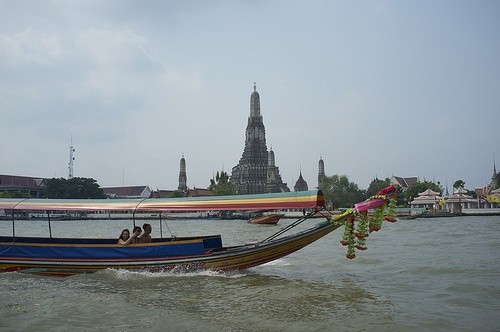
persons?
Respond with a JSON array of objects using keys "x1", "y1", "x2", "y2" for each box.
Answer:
[
  {"x1": 138, "y1": 223, "x2": 152, "y2": 243},
  {"x1": 117, "y1": 229, "x2": 139, "y2": 245},
  {"x1": 130, "y1": 226, "x2": 141, "y2": 244}
]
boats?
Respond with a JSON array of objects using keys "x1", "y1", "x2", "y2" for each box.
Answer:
[
  {"x1": 245, "y1": 209, "x2": 285, "y2": 226},
  {"x1": 0, "y1": 183, "x2": 405, "y2": 274}
]
